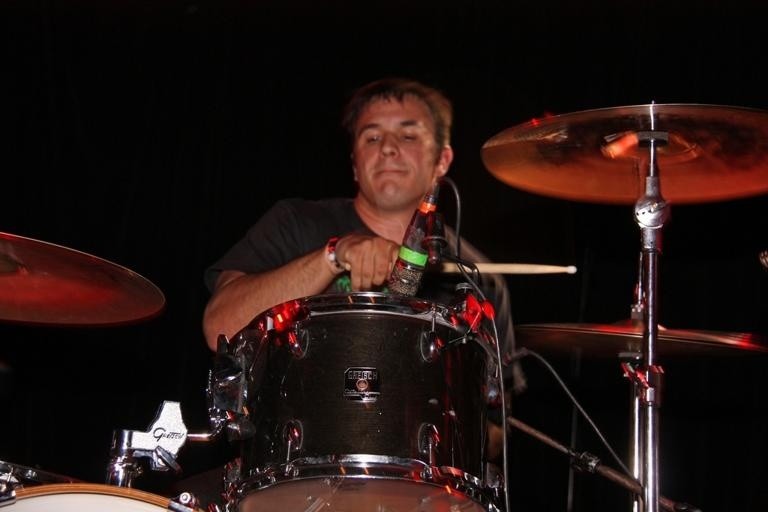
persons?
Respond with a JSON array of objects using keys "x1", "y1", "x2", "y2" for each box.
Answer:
[{"x1": 201, "y1": 78, "x2": 526, "y2": 486}]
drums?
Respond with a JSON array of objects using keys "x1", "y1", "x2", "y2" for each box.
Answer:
[
  {"x1": 208, "y1": 291, "x2": 503, "y2": 510},
  {"x1": 0, "y1": 484, "x2": 221, "y2": 512}
]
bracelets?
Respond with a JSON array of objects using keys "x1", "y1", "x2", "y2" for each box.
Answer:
[{"x1": 326, "y1": 235, "x2": 344, "y2": 272}]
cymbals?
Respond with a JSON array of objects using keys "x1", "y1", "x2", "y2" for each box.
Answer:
[
  {"x1": 517, "y1": 324, "x2": 764, "y2": 352},
  {"x1": 482, "y1": 104, "x2": 768, "y2": 202},
  {"x1": 0, "y1": 231, "x2": 165, "y2": 323}
]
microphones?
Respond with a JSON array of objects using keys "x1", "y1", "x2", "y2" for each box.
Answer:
[{"x1": 388, "y1": 190, "x2": 439, "y2": 297}]
